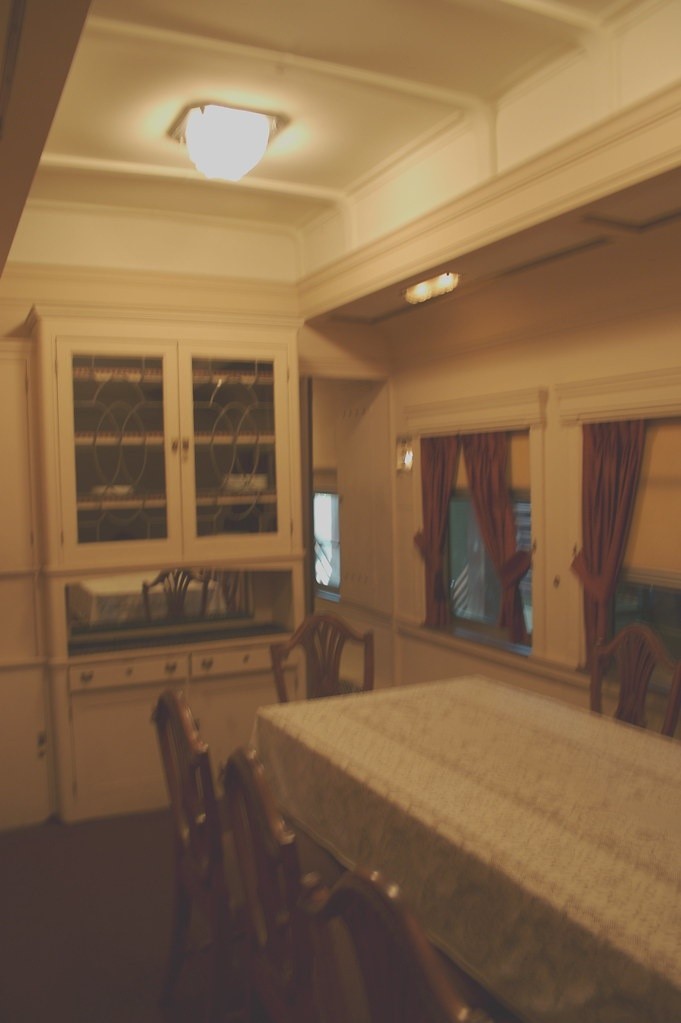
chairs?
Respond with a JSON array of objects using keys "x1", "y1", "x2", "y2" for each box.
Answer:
[
  {"x1": 142, "y1": 567, "x2": 255, "y2": 622},
  {"x1": 156, "y1": 612, "x2": 681, "y2": 1023}
]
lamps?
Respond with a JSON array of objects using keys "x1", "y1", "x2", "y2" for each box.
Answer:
[{"x1": 168, "y1": 99, "x2": 290, "y2": 183}]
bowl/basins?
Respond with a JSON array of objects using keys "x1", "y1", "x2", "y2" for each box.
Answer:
[
  {"x1": 224, "y1": 474, "x2": 267, "y2": 496},
  {"x1": 88, "y1": 484, "x2": 133, "y2": 498}
]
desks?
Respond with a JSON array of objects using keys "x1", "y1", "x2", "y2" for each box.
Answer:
[
  {"x1": 70, "y1": 573, "x2": 230, "y2": 626},
  {"x1": 251, "y1": 675, "x2": 680, "y2": 1022}
]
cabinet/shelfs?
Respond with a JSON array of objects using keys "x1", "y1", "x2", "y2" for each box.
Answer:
[{"x1": 21, "y1": 303, "x2": 307, "y2": 825}]
